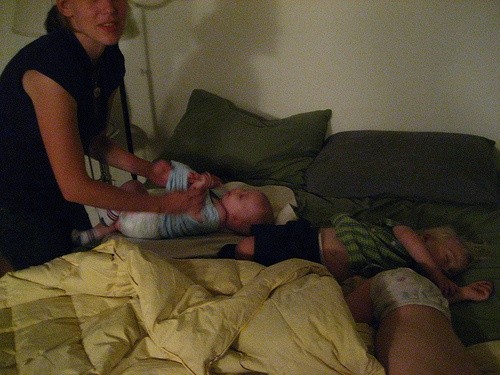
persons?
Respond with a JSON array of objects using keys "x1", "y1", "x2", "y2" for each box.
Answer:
[
  {"x1": 0, "y1": 0, "x2": 206, "y2": 272},
  {"x1": 81, "y1": 172, "x2": 275, "y2": 246},
  {"x1": 216, "y1": 218, "x2": 492, "y2": 300},
  {"x1": 346, "y1": 268, "x2": 481, "y2": 375}
]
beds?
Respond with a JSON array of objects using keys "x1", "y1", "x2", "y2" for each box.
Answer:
[{"x1": 0, "y1": 88, "x2": 500, "y2": 375}]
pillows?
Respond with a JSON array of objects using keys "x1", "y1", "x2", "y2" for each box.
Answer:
[
  {"x1": 144, "y1": 89, "x2": 332, "y2": 191},
  {"x1": 306, "y1": 130, "x2": 497, "y2": 206}
]
